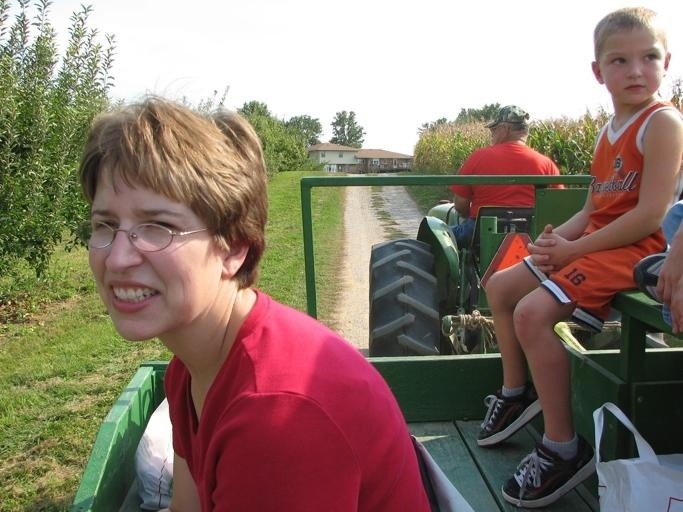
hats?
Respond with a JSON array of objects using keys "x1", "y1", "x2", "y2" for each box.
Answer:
[{"x1": 485, "y1": 106, "x2": 530, "y2": 129}]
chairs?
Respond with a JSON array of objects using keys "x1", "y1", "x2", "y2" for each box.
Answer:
[{"x1": 456, "y1": 204, "x2": 535, "y2": 319}]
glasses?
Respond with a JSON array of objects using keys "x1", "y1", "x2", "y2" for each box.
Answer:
[{"x1": 79, "y1": 220, "x2": 211, "y2": 252}]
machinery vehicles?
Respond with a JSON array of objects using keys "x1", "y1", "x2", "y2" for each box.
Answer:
[{"x1": 69, "y1": 174, "x2": 683, "y2": 510}]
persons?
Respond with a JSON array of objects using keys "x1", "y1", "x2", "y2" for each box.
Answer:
[
  {"x1": 656, "y1": 221, "x2": 682, "y2": 336},
  {"x1": 76, "y1": 94, "x2": 432, "y2": 511},
  {"x1": 468, "y1": 4, "x2": 682, "y2": 512},
  {"x1": 447, "y1": 102, "x2": 565, "y2": 254}
]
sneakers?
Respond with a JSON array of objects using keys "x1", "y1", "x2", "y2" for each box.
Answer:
[
  {"x1": 633, "y1": 254, "x2": 669, "y2": 303},
  {"x1": 502, "y1": 433, "x2": 601, "y2": 508},
  {"x1": 477, "y1": 384, "x2": 542, "y2": 446}
]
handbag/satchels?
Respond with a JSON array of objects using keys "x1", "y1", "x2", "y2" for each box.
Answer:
[{"x1": 593, "y1": 402, "x2": 683, "y2": 512}]
